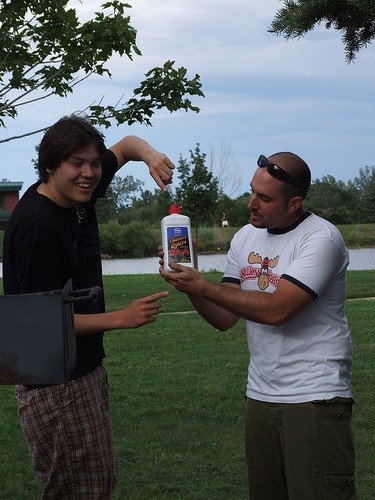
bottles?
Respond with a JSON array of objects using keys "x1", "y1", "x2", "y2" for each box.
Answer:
[{"x1": 160, "y1": 203, "x2": 194, "y2": 272}]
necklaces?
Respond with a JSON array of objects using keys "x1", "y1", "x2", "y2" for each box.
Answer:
[{"x1": 47, "y1": 184, "x2": 78, "y2": 249}]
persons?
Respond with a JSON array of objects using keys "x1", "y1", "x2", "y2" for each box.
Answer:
[
  {"x1": 158, "y1": 152, "x2": 357, "y2": 500},
  {"x1": 3, "y1": 115, "x2": 175, "y2": 500},
  {"x1": 221, "y1": 211, "x2": 229, "y2": 228}
]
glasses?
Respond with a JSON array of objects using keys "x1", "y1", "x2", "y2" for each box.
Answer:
[{"x1": 257, "y1": 155, "x2": 307, "y2": 193}]
